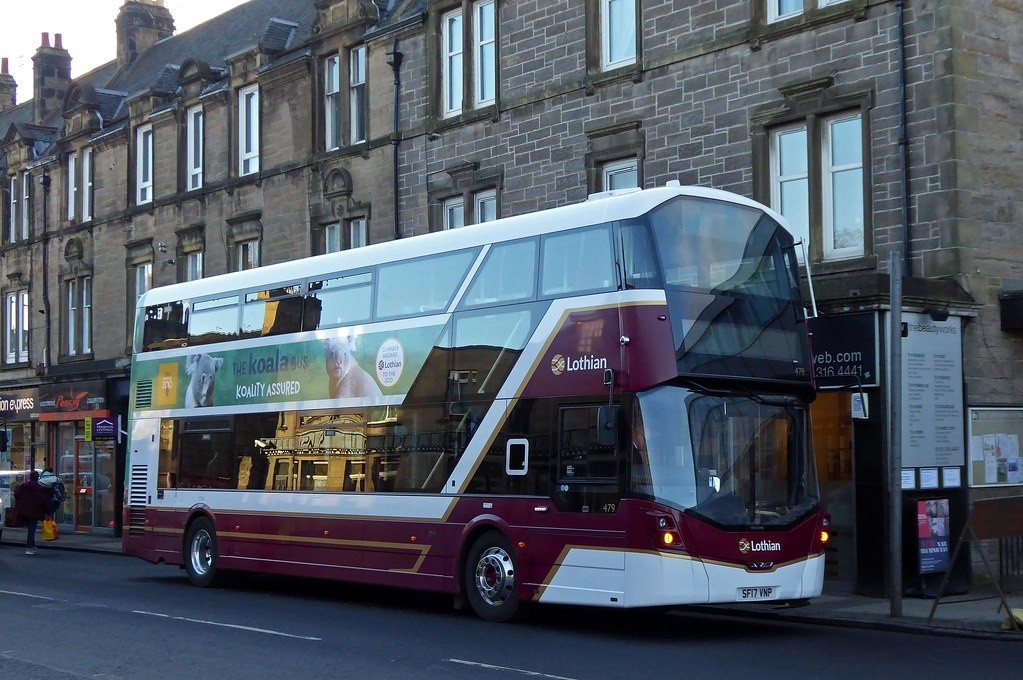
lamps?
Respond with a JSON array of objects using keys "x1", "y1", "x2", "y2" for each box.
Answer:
[{"x1": 930, "y1": 302, "x2": 950, "y2": 321}]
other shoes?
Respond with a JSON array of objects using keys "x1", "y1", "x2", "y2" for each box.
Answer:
[
  {"x1": 25, "y1": 548, "x2": 36, "y2": 555},
  {"x1": 32, "y1": 547, "x2": 38, "y2": 552}
]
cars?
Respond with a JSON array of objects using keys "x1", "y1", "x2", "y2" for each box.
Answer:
[{"x1": 58, "y1": 452, "x2": 113, "y2": 528}]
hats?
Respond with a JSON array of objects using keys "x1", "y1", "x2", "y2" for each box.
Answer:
[{"x1": 29, "y1": 471, "x2": 39, "y2": 478}]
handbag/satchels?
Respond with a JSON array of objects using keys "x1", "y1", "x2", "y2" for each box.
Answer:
[
  {"x1": 40, "y1": 510, "x2": 60, "y2": 542},
  {"x1": 52, "y1": 478, "x2": 65, "y2": 503}
]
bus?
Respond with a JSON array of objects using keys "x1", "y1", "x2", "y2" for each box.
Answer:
[{"x1": 121, "y1": 177, "x2": 873, "y2": 625}]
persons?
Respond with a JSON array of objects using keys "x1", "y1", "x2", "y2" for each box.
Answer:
[
  {"x1": 14, "y1": 470, "x2": 45, "y2": 554},
  {"x1": 926, "y1": 501, "x2": 944, "y2": 517},
  {"x1": 633, "y1": 425, "x2": 650, "y2": 485},
  {"x1": 38, "y1": 467, "x2": 61, "y2": 520}
]
